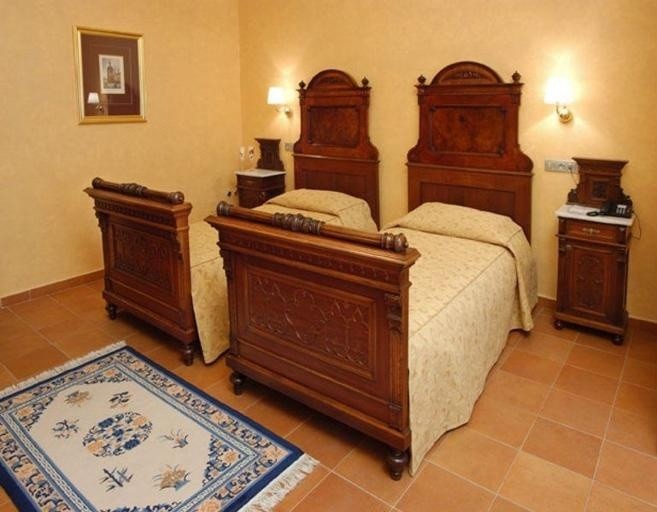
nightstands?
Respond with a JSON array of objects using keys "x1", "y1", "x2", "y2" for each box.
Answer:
[{"x1": 554, "y1": 203, "x2": 636, "y2": 345}]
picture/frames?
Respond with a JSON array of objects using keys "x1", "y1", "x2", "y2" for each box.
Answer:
[{"x1": 73, "y1": 25, "x2": 148, "y2": 125}]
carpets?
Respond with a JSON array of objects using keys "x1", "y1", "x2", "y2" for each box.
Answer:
[{"x1": 0, "y1": 341, "x2": 320, "y2": 511}]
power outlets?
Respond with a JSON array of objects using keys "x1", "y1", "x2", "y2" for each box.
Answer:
[{"x1": 554, "y1": 161, "x2": 576, "y2": 176}]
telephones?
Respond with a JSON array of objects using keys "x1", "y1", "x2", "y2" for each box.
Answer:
[{"x1": 604, "y1": 198, "x2": 632, "y2": 218}]
